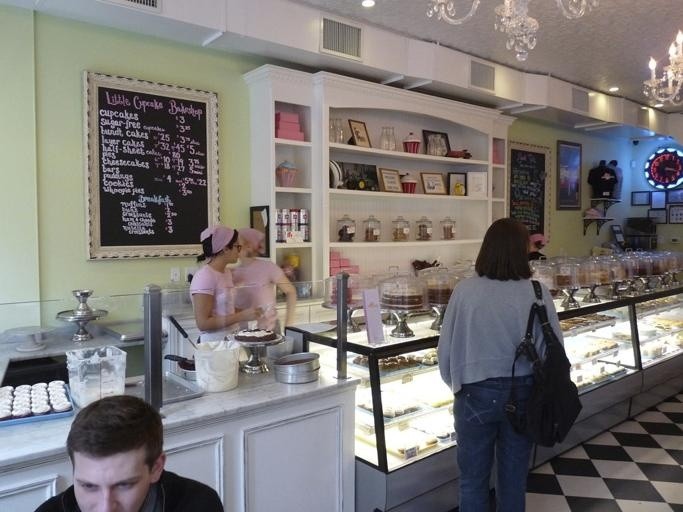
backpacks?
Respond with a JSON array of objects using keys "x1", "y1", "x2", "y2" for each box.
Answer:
[{"x1": 505, "y1": 280, "x2": 582, "y2": 447}]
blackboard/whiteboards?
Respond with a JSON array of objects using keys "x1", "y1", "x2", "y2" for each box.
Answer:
[
  {"x1": 507, "y1": 140, "x2": 552, "y2": 243},
  {"x1": 82, "y1": 70, "x2": 221, "y2": 261}
]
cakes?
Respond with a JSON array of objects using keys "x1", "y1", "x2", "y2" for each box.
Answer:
[
  {"x1": 381, "y1": 251, "x2": 654, "y2": 310},
  {"x1": 559, "y1": 295, "x2": 683, "y2": 390}
]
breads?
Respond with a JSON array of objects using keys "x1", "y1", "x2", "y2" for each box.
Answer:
[{"x1": 352, "y1": 351, "x2": 439, "y2": 375}]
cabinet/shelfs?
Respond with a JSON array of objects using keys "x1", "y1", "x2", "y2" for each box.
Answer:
[
  {"x1": 274, "y1": 301, "x2": 336, "y2": 337},
  {"x1": 241, "y1": 63, "x2": 318, "y2": 298},
  {"x1": 318, "y1": 70, "x2": 492, "y2": 296},
  {"x1": 283, "y1": 296, "x2": 643, "y2": 510},
  {"x1": 580, "y1": 197, "x2": 622, "y2": 237},
  {"x1": 161, "y1": 314, "x2": 200, "y2": 376},
  {"x1": 631, "y1": 281, "x2": 682, "y2": 417},
  {"x1": 492, "y1": 109, "x2": 517, "y2": 224}
]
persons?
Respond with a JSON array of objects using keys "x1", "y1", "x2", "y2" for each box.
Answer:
[
  {"x1": 190, "y1": 226, "x2": 264, "y2": 343},
  {"x1": 228, "y1": 228, "x2": 297, "y2": 334},
  {"x1": 35, "y1": 396, "x2": 223, "y2": 511},
  {"x1": 437, "y1": 218, "x2": 564, "y2": 512},
  {"x1": 529, "y1": 230, "x2": 548, "y2": 261}
]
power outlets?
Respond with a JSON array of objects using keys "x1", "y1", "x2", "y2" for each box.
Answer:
[{"x1": 184, "y1": 267, "x2": 194, "y2": 284}]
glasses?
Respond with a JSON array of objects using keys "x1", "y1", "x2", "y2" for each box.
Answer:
[{"x1": 232, "y1": 244, "x2": 241, "y2": 252}]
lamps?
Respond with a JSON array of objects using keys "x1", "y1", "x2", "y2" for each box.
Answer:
[
  {"x1": 425, "y1": 1, "x2": 600, "y2": 61},
  {"x1": 641, "y1": 29, "x2": 683, "y2": 115}
]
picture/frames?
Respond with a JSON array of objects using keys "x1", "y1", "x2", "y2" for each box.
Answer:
[
  {"x1": 422, "y1": 130, "x2": 451, "y2": 156},
  {"x1": 631, "y1": 191, "x2": 682, "y2": 227},
  {"x1": 554, "y1": 138, "x2": 583, "y2": 212},
  {"x1": 346, "y1": 119, "x2": 371, "y2": 147},
  {"x1": 378, "y1": 167, "x2": 402, "y2": 191},
  {"x1": 447, "y1": 173, "x2": 466, "y2": 196},
  {"x1": 419, "y1": 172, "x2": 446, "y2": 195}
]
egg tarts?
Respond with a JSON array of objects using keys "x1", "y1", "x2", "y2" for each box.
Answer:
[{"x1": 1, "y1": 380, "x2": 72, "y2": 420}]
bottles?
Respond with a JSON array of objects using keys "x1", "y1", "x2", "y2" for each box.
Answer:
[
  {"x1": 380, "y1": 125, "x2": 396, "y2": 150},
  {"x1": 336, "y1": 214, "x2": 356, "y2": 242},
  {"x1": 439, "y1": 216, "x2": 456, "y2": 239},
  {"x1": 362, "y1": 215, "x2": 382, "y2": 242},
  {"x1": 276, "y1": 208, "x2": 310, "y2": 241},
  {"x1": 329, "y1": 118, "x2": 344, "y2": 144},
  {"x1": 391, "y1": 215, "x2": 411, "y2": 242},
  {"x1": 415, "y1": 215, "x2": 433, "y2": 241}
]
